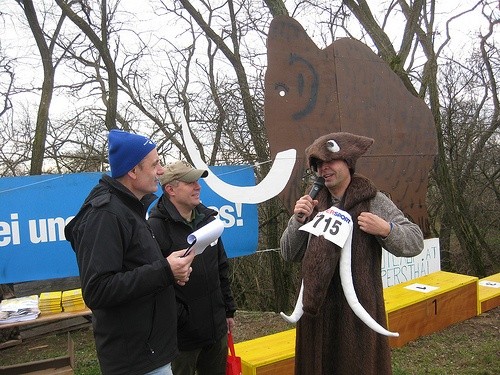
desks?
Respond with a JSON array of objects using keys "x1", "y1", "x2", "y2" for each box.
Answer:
[{"x1": 0, "y1": 304, "x2": 93, "y2": 342}]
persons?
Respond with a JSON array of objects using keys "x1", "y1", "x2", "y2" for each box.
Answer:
[
  {"x1": 278, "y1": 132, "x2": 424, "y2": 375},
  {"x1": 63, "y1": 129, "x2": 197, "y2": 375},
  {"x1": 147, "y1": 161, "x2": 239, "y2": 375}
]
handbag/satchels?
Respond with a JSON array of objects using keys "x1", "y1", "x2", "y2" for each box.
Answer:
[{"x1": 227, "y1": 333, "x2": 243, "y2": 375}]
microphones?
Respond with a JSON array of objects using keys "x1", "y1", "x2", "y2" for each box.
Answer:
[{"x1": 297, "y1": 176, "x2": 325, "y2": 218}]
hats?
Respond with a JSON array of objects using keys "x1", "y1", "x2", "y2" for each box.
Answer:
[
  {"x1": 108, "y1": 129, "x2": 156, "y2": 178},
  {"x1": 305, "y1": 132, "x2": 374, "y2": 178},
  {"x1": 156, "y1": 160, "x2": 209, "y2": 186}
]
